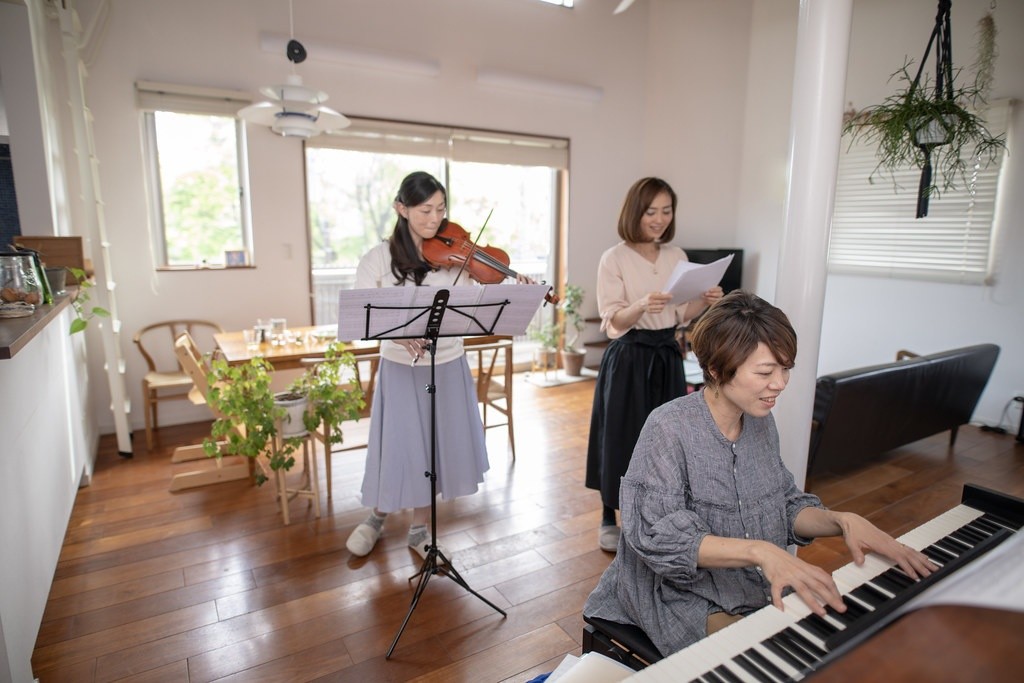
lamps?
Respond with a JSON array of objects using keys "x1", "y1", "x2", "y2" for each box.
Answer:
[{"x1": 237, "y1": 0, "x2": 351, "y2": 141}]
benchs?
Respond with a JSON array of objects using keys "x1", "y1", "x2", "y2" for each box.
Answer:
[{"x1": 811, "y1": 341, "x2": 1000, "y2": 478}]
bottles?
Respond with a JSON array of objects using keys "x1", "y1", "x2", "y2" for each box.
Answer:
[{"x1": 0, "y1": 251, "x2": 44, "y2": 306}]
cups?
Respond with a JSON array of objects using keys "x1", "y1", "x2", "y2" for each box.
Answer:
[
  {"x1": 224, "y1": 249, "x2": 246, "y2": 266},
  {"x1": 270, "y1": 318, "x2": 287, "y2": 345},
  {"x1": 243, "y1": 330, "x2": 262, "y2": 350},
  {"x1": 254, "y1": 325, "x2": 271, "y2": 344},
  {"x1": 45, "y1": 265, "x2": 67, "y2": 295}
]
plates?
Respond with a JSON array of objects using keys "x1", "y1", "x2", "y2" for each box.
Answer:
[{"x1": 311, "y1": 330, "x2": 337, "y2": 341}]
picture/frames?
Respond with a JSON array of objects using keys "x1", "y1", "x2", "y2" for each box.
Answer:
[{"x1": 223, "y1": 249, "x2": 250, "y2": 268}]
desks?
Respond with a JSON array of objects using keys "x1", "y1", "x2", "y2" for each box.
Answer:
[{"x1": 211, "y1": 320, "x2": 514, "y2": 486}]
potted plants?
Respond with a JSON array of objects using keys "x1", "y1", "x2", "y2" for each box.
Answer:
[
  {"x1": 199, "y1": 340, "x2": 367, "y2": 490},
  {"x1": 45, "y1": 266, "x2": 112, "y2": 335},
  {"x1": 841, "y1": 0, "x2": 1024, "y2": 200},
  {"x1": 560, "y1": 286, "x2": 588, "y2": 378},
  {"x1": 527, "y1": 319, "x2": 565, "y2": 363}
]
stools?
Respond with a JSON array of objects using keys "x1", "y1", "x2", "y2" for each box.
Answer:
[{"x1": 581, "y1": 613, "x2": 662, "y2": 671}]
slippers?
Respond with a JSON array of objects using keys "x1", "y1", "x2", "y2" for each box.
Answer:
[
  {"x1": 346, "y1": 521, "x2": 385, "y2": 557},
  {"x1": 408, "y1": 537, "x2": 452, "y2": 566},
  {"x1": 598, "y1": 523, "x2": 621, "y2": 552}
]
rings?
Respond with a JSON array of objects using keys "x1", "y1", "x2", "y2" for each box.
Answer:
[{"x1": 900, "y1": 543, "x2": 904, "y2": 548}]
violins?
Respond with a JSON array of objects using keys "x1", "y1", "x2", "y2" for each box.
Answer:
[{"x1": 415, "y1": 218, "x2": 562, "y2": 307}]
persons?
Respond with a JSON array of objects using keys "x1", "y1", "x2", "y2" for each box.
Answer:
[
  {"x1": 586, "y1": 175, "x2": 725, "y2": 550},
  {"x1": 583, "y1": 287, "x2": 940, "y2": 660},
  {"x1": 345, "y1": 170, "x2": 539, "y2": 570}
]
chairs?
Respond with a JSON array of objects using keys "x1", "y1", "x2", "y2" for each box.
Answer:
[
  {"x1": 297, "y1": 352, "x2": 381, "y2": 494},
  {"x1": 135, "y1": 319, "x2": 226, "y2": 451},
  {"x1": 463, "y1": 339, "x2": 515, "y2": 455},
  {"x1": 167, "y1": 331, "x2": 267, "y2": 492}
]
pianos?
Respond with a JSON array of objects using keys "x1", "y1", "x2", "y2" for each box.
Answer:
[{"x1": 611, "y1": 473, "x2": 1024, "y2": 683}]
